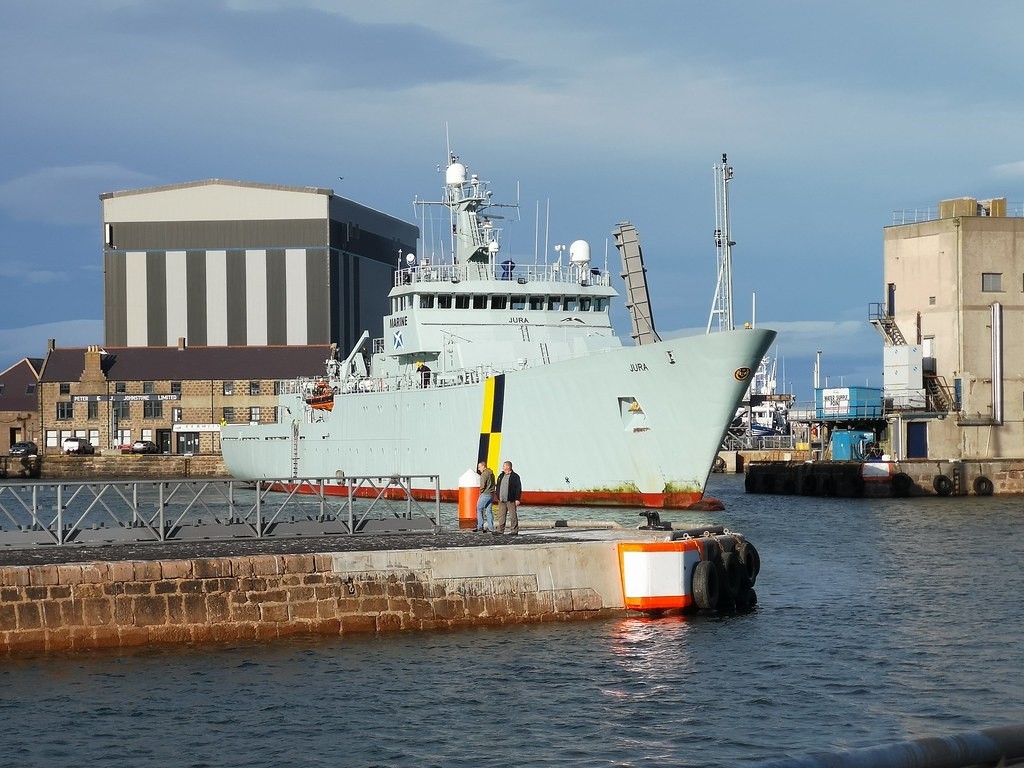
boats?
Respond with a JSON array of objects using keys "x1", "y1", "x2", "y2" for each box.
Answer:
[
  {"x1": 306, "y1": 390, "x2": 335, "y2": 411},
  {"x1": 219, "y1": 119, "x2": 778, "y2": 514}
]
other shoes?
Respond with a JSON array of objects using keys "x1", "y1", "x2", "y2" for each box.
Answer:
[
  {"x1": 493, "y1": 531, "x2": 504, "y2": 535},
  {"x1": 508, "y1": 532, "x2": 518, "y2": 535}
]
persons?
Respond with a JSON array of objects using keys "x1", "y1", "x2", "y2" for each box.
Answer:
[
  {"x1": 416, "y1": 362, "x2": 431, "y2": 389},
  {"x1": 472, "y1": 462, "x2": 495, "y2": 532},
  {"x1": 492, "y1": 461, "x2": 522, "y2": 535}
]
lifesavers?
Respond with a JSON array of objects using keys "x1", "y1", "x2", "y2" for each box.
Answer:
[
  {"x1": 932, "y1": 475, "x2": 954, "y2": 496},
  {"x1": 691, "y1": 540, "x2": 762, "y2": 608},
  {"x1": 761, "y1": 474, "x2": 775, "y2": 494},
  {"x1": 973, "y1": 474, "x2": 994, "y2": 495},
  {"x1": 804, "y1": 475, "x2": 819, "y2": 494},
  {"x1": 841, "y1": 471, "x2": 864, "y2": 498},
  {"x1": 745, "y1": 475, "x2": 756, "y2": 493},
  {"x1": 784, "y1": 479, "x2": 798, "y2": 495},
  {"x1": 712, "y1": 457, "x2": 725, "y2": 473},
  {"x1": 891, "y1": 471, "x2": 916, "y2": 495}
]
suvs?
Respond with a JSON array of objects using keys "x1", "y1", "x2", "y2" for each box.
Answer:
[{"x1": 63, "y1": 436, "x2": 94, "y2": 457}]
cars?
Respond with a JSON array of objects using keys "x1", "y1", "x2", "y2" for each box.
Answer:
[
  {"x1": 130, "y1": 440, "x2": 159, "y2": 455},
  {"x1": 9, "y1": 440, "x2": 38, "y2": 458}
]
